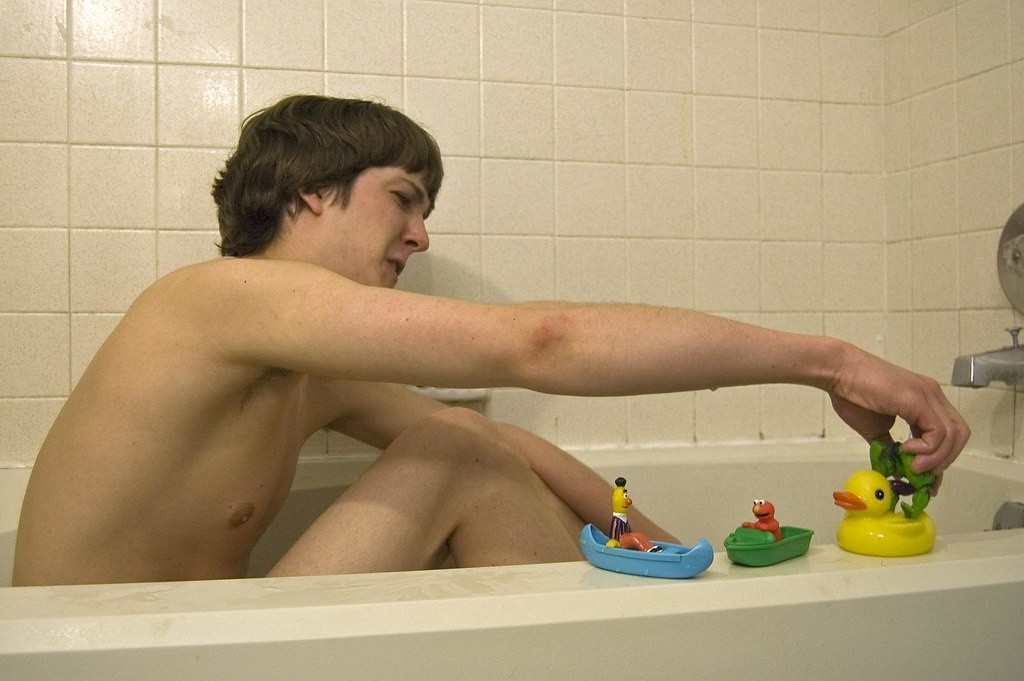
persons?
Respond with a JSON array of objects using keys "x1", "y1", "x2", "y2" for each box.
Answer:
[{"x1": 12, "y1": 95, "x2": 971, "y2": 586}]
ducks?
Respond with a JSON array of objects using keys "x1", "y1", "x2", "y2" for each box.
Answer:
[{"x1": 832, "y1": 470, "x2": 937, "y2": 557}]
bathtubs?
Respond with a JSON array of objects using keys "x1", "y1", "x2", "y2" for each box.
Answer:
[{"x1": 0, "y1": 434, "x2": 1023, "y2": 681}]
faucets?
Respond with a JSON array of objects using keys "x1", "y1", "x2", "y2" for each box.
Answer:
[{"x1": 950, "y1": 328, "x2": 1024, "y2": 388}]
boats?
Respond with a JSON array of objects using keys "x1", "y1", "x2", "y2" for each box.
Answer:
[
  {"x1": 579, "y1": 522, "x2": 715, "y2": 579},
  {"x1": 723, "y1": 523, "x2": 816, "y2": 567}
]
